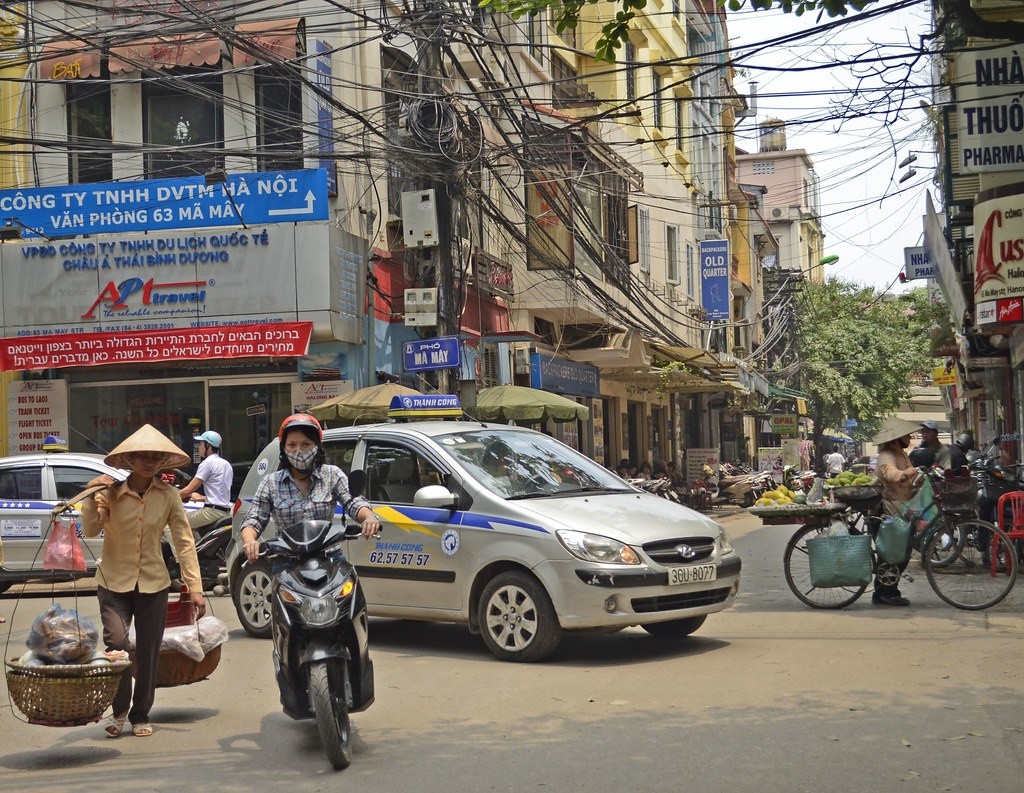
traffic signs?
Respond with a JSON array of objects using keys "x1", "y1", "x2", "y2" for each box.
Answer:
[{"x1": 0, "y1": 168, "x2": 330, "y2": 238}]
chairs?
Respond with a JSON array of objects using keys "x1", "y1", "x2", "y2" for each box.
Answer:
[
  {"x1": 375, "y1": 456, "x2": 417, "y2": 500},
  {"x1": 990, "y1": 490, "x2": 1024, "y2": 577}
]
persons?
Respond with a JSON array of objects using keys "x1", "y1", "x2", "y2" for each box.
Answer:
[
  {"x1": 606, "y1": 459, "x2": 677, "y2": 481},
  {"x1": 163, "y1": 431, "x2": 233, "y2": 579},
  {"x1": 944, "y1": 434, "x2": 974, "y2": 476},
  {"x1": 907, "y1": 419, "x2": 951, "y2": 495},
  {"x1": 988, "y1": 437, "x2": 1002, "y2": 464},
  {"x1": 241, "y1": 413, "x2": 380, "y2": 573},
  {"x1": 81, "y1": 424, "x2": 206, "y2": 737},
  {"x1": 871, "y1": 416, "x2": 925, "y2": 606},
  {"x1": 827, "y1": 445, "x2": 847, "y2": 478},
  {"x1": 481, "y1": 442, "x2": 562, "y2": 495}
]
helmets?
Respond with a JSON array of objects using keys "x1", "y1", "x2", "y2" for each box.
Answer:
[
  {"x1": 279, "y1": 413, "x2": 322, "y2": 447},
  {"x1": 909, "y1": 445, "x2": 935, "y2": 470},
  {"x1": 193, "y1": 430, "x2": 222, "y2": 449},
  {"x1": 956, "y1": 433, "x2": 974, "y2": 450}
]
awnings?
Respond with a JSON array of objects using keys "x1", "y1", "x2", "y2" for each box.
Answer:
[
  {"x1": 650, "y1": 344, "x2": 769, "y2": 398},
  {"x1": 808, "y1": 426, "x2": 854, "y2": 471}
]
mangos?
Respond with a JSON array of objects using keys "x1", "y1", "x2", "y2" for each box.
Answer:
[
  {"x1": 754, "y1": 485, "x2": 807, "y2": 506},
  {"x1": 826, "y1": 471, "x2": 873, "y2": 485}
]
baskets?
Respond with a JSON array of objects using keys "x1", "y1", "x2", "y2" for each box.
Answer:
[
  {"x1": 125, "y1": 642, "x2": 221, "y2": 687},
  {"x1": 6, "y1": 670, "x2": 121, "y2": 727},
  {"x1": 3, "y1": 656, "x2": 134, "y2": 677},
  {"x1": 939, "y1": 476, "x2": 978, "y2": 516}
]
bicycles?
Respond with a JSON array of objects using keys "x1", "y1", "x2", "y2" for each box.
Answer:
[{"x1": 784, "y1": 465, "x2": 1018, "y2": 610}]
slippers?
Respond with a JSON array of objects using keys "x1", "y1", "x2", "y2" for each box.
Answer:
[
  {"x1": 132, "y1": 722, "x2": 152, "y2": 736},
  {"x1": 104, "y1": 714, "x2": 126, "y2": 737}
]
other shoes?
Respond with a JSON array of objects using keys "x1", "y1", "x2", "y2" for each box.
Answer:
[
  {"x1": 169, "y1": 564, "x2": 181, "y2": 580},
  {"x1": 875, "y1": 591, "x2": 910, "y2": 606}
]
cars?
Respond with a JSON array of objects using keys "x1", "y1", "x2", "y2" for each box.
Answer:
[
  {"x1": 914, "y1": 437, "x2": 1023, "y2": 572},
  {"x1": 0, "y1": 436, "x2": 232, "y2": 594},
  {"x1": 846, "y1": 455, "x2": 877, "y2": 475},
  {"x1": 225, "y1": 393, "x2": 741, "y2": 663}
]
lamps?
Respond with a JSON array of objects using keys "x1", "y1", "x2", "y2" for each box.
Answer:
[
  {"x1": 0, "y1": 216, "x2": 52, "y2": 242},
  {"x1": 204, "y1": 166, "x2": 249, "y2": 230},
  {"x1": 899, "y1": 164, "x2": 941, "y2": 183},
  {"x1": 899, "y1": 144, "x2": 938, "y2": 168}
]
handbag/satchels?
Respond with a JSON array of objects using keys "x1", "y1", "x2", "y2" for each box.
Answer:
[
  {"x1": 43, "y1": 522, "x2": 87, "y2": 573},
  {"x1": 25, "y1": 602, "x2": 99, "y2": 664},
  {"x1": 805, "y1": 519, "x2": 873, "y2": 587},
  {"x1": 874, "y1": 511, "x2": 912, "y2": 565}
]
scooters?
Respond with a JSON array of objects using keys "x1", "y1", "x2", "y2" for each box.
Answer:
[
  {"x1": 625, "y1": 459, "x2": 817, "y2": 508},
  {"x1": 159, "y1": 488, "x2": 232, "y2": 593},
  {"x1": 240, "y1": 469, "x2": 383, "y2": 770}
]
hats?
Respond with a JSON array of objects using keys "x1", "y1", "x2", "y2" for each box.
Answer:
[
  {"x1": 104, "y1": 423, "x2": 192, "y2": 469},
  {"x1": 871, "y1": 414, "x2": 924, "y2": 446},
  {"x1": 919, "y1": 419, "x2": 939, "y2": 431}
]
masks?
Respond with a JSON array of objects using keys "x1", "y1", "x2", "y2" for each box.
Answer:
[{"x1": 283, "y1": 445, "x2": 319, "y2": 469}]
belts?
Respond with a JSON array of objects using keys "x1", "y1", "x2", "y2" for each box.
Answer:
[{"x1": 204, "y1": 503, "x2": 230, "y2": 512}]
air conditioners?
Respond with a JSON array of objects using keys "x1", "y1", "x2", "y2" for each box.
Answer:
[
  {"x1": 461, "y1": 238, "x2": 472, "y2": 276},
  {"x1": 770, "y1": 205, "x2": 790, "y2": 219},
  {"x1": 728, "y1": 204, "x2": 738, "y2": 221}
]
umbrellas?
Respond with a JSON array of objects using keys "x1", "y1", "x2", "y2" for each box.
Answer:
[
  {"x1": 476, "y1": 383, "x2": 589, "y2": 428},
  {"x1": 300, "y1": 380, "x2": 424, "y2": 420}
]
